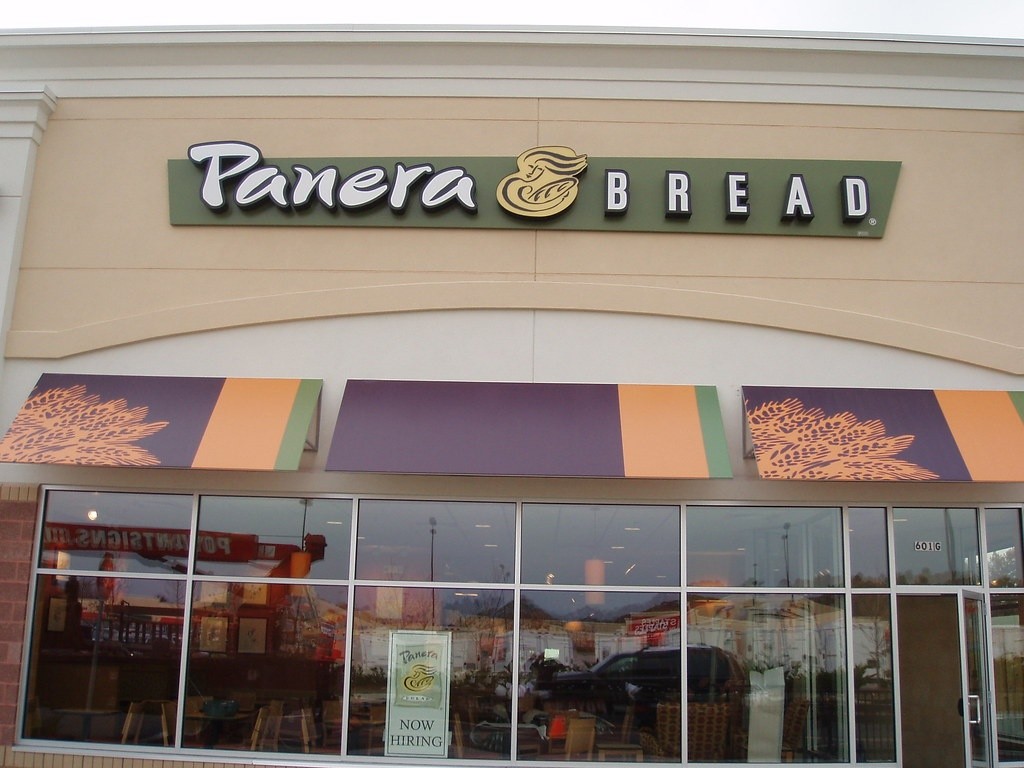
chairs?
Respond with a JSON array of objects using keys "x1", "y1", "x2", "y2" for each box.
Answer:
[
  {"x1": 25, "y1": 683, "x2": 645, "y2": 766},
  {"x1": 636, "y1": 698, "x2": 810, "y2": 764}
]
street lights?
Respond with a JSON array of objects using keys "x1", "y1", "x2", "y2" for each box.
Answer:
[
  {"x1": 299, "y1": 498, "x2": 313, "y2": 551},
  {"x1": 781, "y1": 520, "x2": 791, "y2": 587},
  {"x1": 427, "y1": 516, "x2": 438, "y2": 630}
]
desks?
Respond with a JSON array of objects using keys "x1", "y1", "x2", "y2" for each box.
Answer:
[
  {"x1": 52, "y1": 707, "x2": 120, "y2": 742},
  {"x1": 471, "y1": 724, "x2": 542, "y2": 761},
  {"x1": 183, "y1": 710, "x2": 253, "y2": 750},
  {"x1": 323, "y1": 718, "x2": 386, "y2": 752}
]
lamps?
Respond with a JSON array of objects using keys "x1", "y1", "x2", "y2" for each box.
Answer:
[
  {"x1": 584, "y1": 508, "x2": 605, "y2": 608},
  {"x1": 289, "y1": 498, "x2": 311, "y2": 599}
]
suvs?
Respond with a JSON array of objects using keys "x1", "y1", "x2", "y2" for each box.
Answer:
[{"x1": 552, "y1": 644, "x2": 733, "y2": 717}]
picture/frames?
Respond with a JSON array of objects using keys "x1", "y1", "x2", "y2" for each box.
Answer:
[
  {"x1": 237, "y1": 576, "x2": 271, "y2": 608},
  {"x1": 237, "y1": 616, "x2": 269, "y2": 655},
  {"x1": 46, "y1": 596, "x2": 68, "y2": 634},
  {"x1": 51, "y1": 549, "x2": 72, "y2": 586}
]
